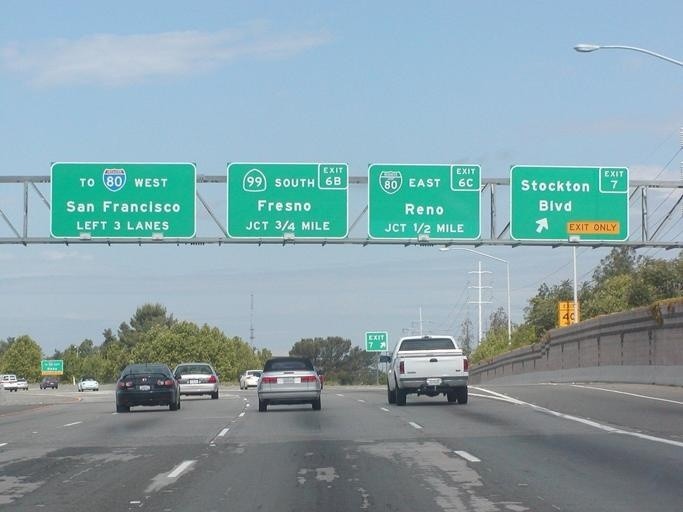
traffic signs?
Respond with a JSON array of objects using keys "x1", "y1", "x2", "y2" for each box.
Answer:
[
  {"x1": 367, "y1": 162, "x2": 481, "y2": 242},
  {"x1": 48, "y1": 162, "x2": 196, "y2": 240},
  {"x1": 223, "y1": 162, "x2": 347, "y2": 241},
  {"x1": 365, "y1": 332, "x2": 387, "y2": 351},
  {"x1": 40, "y1": 359, "x2": 63, "y2": 376},
  {"x1": 509, "y1": 165, "x2": 629, "y2": 244}
]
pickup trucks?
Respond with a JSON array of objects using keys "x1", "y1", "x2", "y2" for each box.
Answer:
[
  {"x1": 380, "y1": 335, "x2": 470, "y2": 405},
  {"x1": 0, "y1": 374, "x2": 17, "y2": 391}
]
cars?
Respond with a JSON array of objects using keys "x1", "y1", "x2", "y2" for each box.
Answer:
[
  {"x1": 39, "y1": 376, "x2": 58, "y2": 389},
  {"x1": 115, "y1": 358, "x2": 180, "y2": 412},
  {"x1": 77, "y1": 376, "x2": 98, "y2": 392},
  {"x1": 16, "y1": 378, "x2": 28, "y2": 391},
  {"x1": 173, "y1": 363, "x2": 219, "y2": 399},
  {"x1": 238, "y1": 355, "x2": 324, "y2": 412}
]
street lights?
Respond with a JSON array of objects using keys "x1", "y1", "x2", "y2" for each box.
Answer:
[
  {"x1": 437, "y1": 247, "x2": 512, "y2": 345},
  {"x1": 572, "y1": 44, "x2": 682, "y2": 68},
  {"x1": 73, "y1": 345, "x2": 78, "y2": 357}
]
